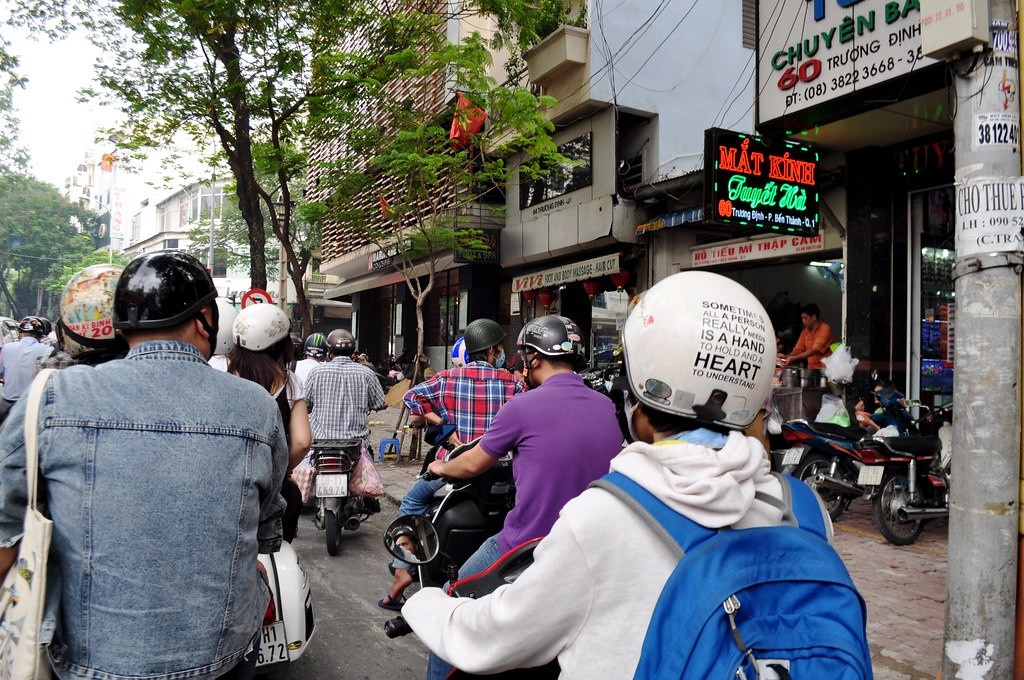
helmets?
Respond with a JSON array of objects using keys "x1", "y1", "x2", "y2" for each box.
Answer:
[
  {"x1": 325, "y1": 329, "x2": 355, "y2": 354},
  {"x1": 111, "y1": 249, "x2": 218, "y2": 337},
  {"x1": 464, "y1": 318, "x2": 508, "y2": 354},
  {"x1": 451, "y1": 336, "x2": 470, "y2": 367},
  {"x1": 20, "y1": 316, "x2": 52, "y2": 334},
  {"x1": 214, "y1": 297, "x2": 239, "y2": 355},
  {"x1": 233, "y1": 302, "x2": 291, "y2": 351},
  {"x1": 393, "y1": 529, "x2": 417, "y2": 548},
  {"x1": 516, "y1": 316, "x2": 586, "y2": 372},
  {"x1": 303, "y1": 333, "x2": 328, "y2": 358},
  {"x1": 60, "y1": 264, "x2": 126, "y2": 360},
  {"x1": 289, "y1": 333, "x2": 303, "y2": 359},
  {"x1": 620, "y1": 269, "x2": 776, "y2": 430},
  {"x1": 55, "y1": 318, "x2": 64, "y2": 343}
]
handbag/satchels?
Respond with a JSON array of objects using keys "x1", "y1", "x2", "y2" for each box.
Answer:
[
  {"x1": 288, "y1": 450, "x2": 315, "y2": 504},
  {"x1": 0, "y1": 368, "x2": 59, "y2": 680},
  {"x1": 813, "y1": 394, "x2": 851, "y2": 427},
  {"x1": 348, "y1": 447, "x2": 383, "y2": 498}
]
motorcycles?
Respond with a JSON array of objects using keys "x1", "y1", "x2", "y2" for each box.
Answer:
[
  {"x1": 309, "y1": 406, "x2": 380, "y2": 558},
  {"x1": 778, "y1": 367, "x2": 931, "y2": 522},
  {"x1": 850, "y1": 398, "x2": 954, "y2": 548}
]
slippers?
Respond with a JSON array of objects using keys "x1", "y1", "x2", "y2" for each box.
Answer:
[{"x1": 378, "y1": 593, "x2": 407, "y2": 611}]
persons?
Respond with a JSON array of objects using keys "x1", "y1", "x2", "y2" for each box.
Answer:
[
  {"x1": 286, "y1": 333, "x2": 328, "y2": 381},
  {"x1": 380, "y1": 318, "x2": 529, "y2": 611},
  {"x1": 785, "y1": 303, "x2": 837, "y2": 370},
  {"x1": 0, "y1": 251, "x2": 289, "y2": 680},
  {"x1": 391, "y1": 526, "x2": 418, "y2": 553},
  {"x1": 426, "y1": 315, "x2": 623, "y2": 680},
  {"x1": 401, "y1": 270, "x2": 835, "y2": 680},
  {"x1": 207, "y1": 299, "x2": 312, "y2": 543},
  {"x1": 304, "y1": 329, "x2": 388, "y2": 512}
]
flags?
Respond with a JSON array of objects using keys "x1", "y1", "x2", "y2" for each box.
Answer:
[{"x1": 450, "y1": 97, "x2": 487, "y2": 150}]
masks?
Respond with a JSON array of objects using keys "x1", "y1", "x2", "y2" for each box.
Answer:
[
  {"x1": 623, "y1": 390, "x2": 641, "y2": 442},
  {"x1": 489, "y1": 345, "x2": 505, "y2": 368}
]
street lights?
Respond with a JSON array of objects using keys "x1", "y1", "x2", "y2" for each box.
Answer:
[{"x1": 78, "y1": 195, "x2": 115, "y2": 265}]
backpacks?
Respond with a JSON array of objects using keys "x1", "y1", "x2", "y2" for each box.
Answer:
[{"x1": 585, "y1": 471, "x2": 873, "y2": 680}]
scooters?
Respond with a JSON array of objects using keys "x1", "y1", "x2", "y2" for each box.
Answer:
[
  {"x1": 381, "y1": 512, "x2": 560, "y2": 680},
  {"x1": 238, "y1": 538, "x2": 319, "y2": 673},
  {"x1": 578, "y1": 362, "x2": 638, "y2": 447},
  {"x1": 415, "y1": 421, "x2": 516, "y2": 590},
  {"x1": 350, "y1": 345, "x2": 436, "y2": 396}
]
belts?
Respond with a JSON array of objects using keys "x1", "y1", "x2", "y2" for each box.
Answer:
[{"x1": 3, "y1": 398, "x2": 17, "y2": 404}]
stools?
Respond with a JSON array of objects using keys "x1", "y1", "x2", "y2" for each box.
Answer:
[{"x1": 379, "y1": 438, "x2": 402, "y2": 462}]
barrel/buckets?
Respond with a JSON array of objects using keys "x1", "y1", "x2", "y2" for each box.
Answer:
[{"x1": 782, "y1": 367, "x2": 819, "y2": 387}]
